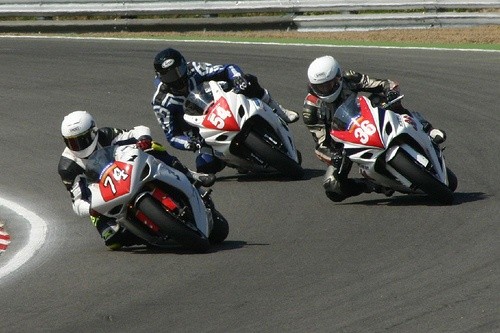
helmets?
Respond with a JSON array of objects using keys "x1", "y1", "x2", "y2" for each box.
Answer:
[
  {"x1": 307, "y1": 55, "x2": 344, "y2": 103},
  {"x1": 153, "y1": 48, "x2": 187, "y2": 90},
  {"x1": 60, "y1": 111, "x2": 99, "y2": 158}
]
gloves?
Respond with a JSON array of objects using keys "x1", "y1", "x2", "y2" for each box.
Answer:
[
  {"x1": 89, "y1": 206, "x2": 100, "y2": 218},
  {"x1": 136, "y1": 136, "x2": 152, "y2": 150},
  {"x1": 189, "y1": 137, "x2": 204, "y2": 152},
  {"x1": 332, "y1": 154, "x2": 343, "y2": 168},
  {"x1": 230, "y1": 75, "x2": 248, "y2": 90},
  {"x1": 386, "y1": 91, "x2": 401, "y2": 107}
]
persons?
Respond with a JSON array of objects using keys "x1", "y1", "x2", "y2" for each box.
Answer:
[
  {"x1": 57, "y1": 110, "x2": 216, "y2": 250},
  {"x1": 150, "y1": 48, "x2": 299, "y2": 175},
  {"x1": 302, "y1": 55, "x2": 415, "y2": 202}
]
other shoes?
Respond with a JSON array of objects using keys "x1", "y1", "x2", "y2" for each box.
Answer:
[
  {"x1": 374, "y1": 184, "x2": 394, "y2": 197},
  {"x1": 425, "y1": 124, "x2": 447, "y2": 143},
  {"x1": 186, "y1": 169, "x2": 216, "y2": 187},
  {"x1": 273, "y1": 104, "x2": 299, "y2": 123}
]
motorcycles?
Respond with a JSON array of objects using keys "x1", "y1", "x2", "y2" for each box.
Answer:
[
  {"x1": 306, "y1": 87, "x2": 457, "y2": 204},
  {"x1": 79, "y1": 140, "x2": 230, "y2": 254},
  {"x1": 181, "y1": 79, "x2": 305, "y2": 179}
]
